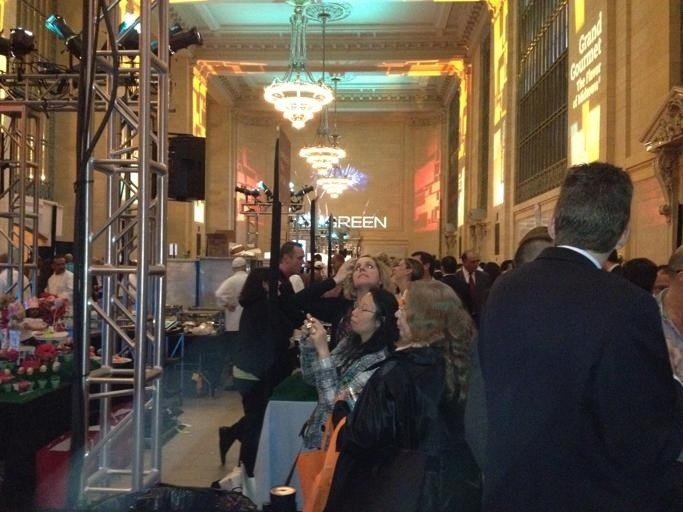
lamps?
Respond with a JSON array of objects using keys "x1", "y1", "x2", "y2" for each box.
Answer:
[
  {"x1": 0, "y1": 14, "x2": 205, "y2": 72},
  {"x1": 235, "y1": 178, "x2": 350, "y2": 242},
  {"x1": 262, "y1": 0, "x2": 353, "y2": 200}
]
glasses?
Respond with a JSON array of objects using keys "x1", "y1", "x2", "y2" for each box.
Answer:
[{"x1": 353, "y1": 301, "x2": 376, "y2": 314}]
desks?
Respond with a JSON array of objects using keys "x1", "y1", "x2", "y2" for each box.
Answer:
[{"x1": 0, "y1": 308, "x2": 176, "y2": 512}]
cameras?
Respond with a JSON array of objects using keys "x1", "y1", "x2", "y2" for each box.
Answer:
[{"x1": 305, "y1": 320, "x2": 324, "y2": 334}]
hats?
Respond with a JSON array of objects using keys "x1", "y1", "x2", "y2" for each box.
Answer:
[{"x1": 231, "y1": 256, "x2": 246, "y2": 268}]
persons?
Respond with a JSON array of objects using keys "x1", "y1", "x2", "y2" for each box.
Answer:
[
  {"x1": 36, "y1": 252, "x2": 137, "y2": 304},
  {"x1": 44, "y1": 255, "x2": 74, "y2": 316},
  {"x1": 0, "y1": 253, "x2": 32, "y2": 298},
  {"x1": 211, "y1": 249, "x2": 683, "y2": 509},
  {"x1": 475, "y1": 162, "x2": 679, "y2": 511}
]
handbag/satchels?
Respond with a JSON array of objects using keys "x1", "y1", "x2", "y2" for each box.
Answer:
[{"x1": 298, "y1": 416, "x2": 346, "y2": 512}]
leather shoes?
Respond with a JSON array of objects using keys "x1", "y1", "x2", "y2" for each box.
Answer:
[{"x1": 219, "y1": 426, "x2": 234, "y2": 465}]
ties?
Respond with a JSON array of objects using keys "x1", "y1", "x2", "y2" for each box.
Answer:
[{"x1": 469, "y1": 274, "x2": 475, "y2": 296}]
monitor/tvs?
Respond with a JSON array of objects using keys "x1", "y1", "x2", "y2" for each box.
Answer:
[{"x1": 151, "y1": 135, "x2": 206, "y2": 203}]
